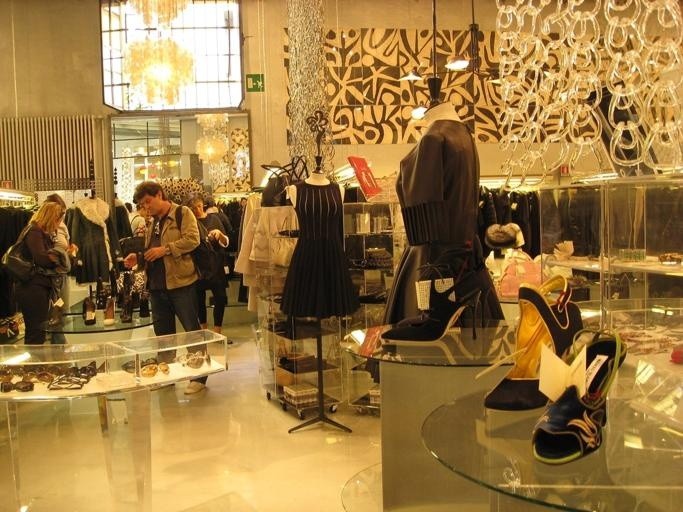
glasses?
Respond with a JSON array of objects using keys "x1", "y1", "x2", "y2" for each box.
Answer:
[
  {"x1": 121, "y1": 357, "x2": 169, "y2": 378},
  {"x1": 176, "y1": 350, "x2": 212, "y2": 370},
  {"x1": 0, "y1": 363, "x2": 97, "y2": 392}
]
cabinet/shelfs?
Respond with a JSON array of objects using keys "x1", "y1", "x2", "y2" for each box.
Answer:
[{"x1": 1, "y1": 202, "x2": 682, "y2": 512}]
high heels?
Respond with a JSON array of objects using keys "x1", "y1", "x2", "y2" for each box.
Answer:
[
  {"x1": 104, "y1": 299, "x2": 116, "y2": 326},
  {"x1": 484, "y1": 275, "x2": 626, "y2": 466},
  {"x1": 380, "y1": 257, "x2": 486, "y2": 343},
  {"x1": 121, "y1": 295, "x2": 134, "y2": 323},
  {"x1": 84, "y1": 299, "x2": 96, "y2": 325},
  {"x1": 140, "y1": 297, "x2": 150, "y2": 317}
]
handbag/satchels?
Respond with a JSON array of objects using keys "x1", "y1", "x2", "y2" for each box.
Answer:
[
  {"x1": 498, "y1": 262, "x2": 548, "y2": 298},
  {"x1": 1, "y1": 241, "x2": 38, "y2": 282},
  {"x1": 272, "y1": 215, "x2": 298, "y2": 268},
  {"x1": 262, "y1": 156, "x2": 310, "y2": 208}
]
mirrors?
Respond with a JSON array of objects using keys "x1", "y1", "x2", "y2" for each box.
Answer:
[{"x1": 104, "y1": 113, "x2": 255, "y2": 226}]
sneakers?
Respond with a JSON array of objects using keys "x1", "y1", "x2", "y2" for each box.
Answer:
[{"x1": 183, "y1": 380, "x2": 206, "y2": 394}]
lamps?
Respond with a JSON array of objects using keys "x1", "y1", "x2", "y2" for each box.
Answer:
[
  {"x1": 196, "y1": 115, "x2": 233, "y2": 167},
  {"x1": 124, "y1": 1, "x2": 194, "y2": 107},
  {"x1": 399, "y1": 0, "x2": 500, "y2": 119}
]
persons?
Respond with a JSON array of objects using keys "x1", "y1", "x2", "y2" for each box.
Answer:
[
  {"x1": 363, "y1": 99, "x2": 505, "y2": 386},
  {"x1": 279, "y1": 171, "x2": 362, "y2": 316},
  {"x1": 37, "y1": 193, "x2": 79, "y2": 368},
  {"x1": 10, "y1": 201, "x2": 65, "y2": 369},
  {"x1": 123, "y1": 197, "x2": 250, "y2": 245},
  {"x1": 180, "y1": 189, "x2": 233, "y2": 345},
  {"x1": 124, "y1": 180, "x2": 211, "y2": 394}
]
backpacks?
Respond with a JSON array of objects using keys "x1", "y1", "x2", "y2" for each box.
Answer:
[{"x1": 189, "y1": 219, "x2": 221, "y2": 280}]
